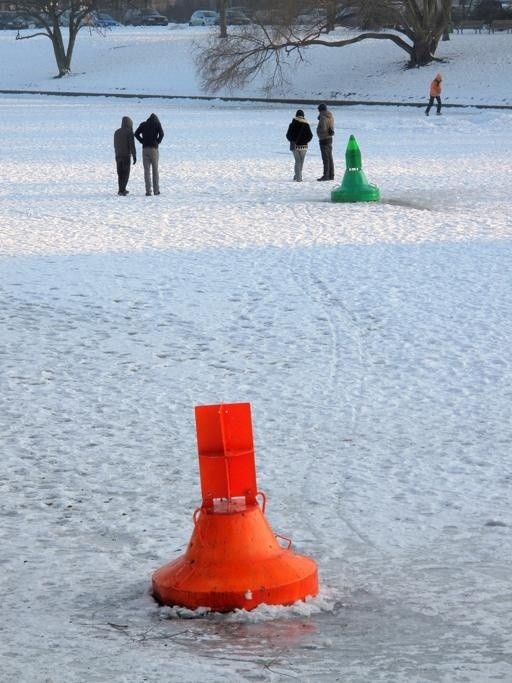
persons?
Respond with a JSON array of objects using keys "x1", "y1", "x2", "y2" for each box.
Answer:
[
  {"x1": 286, "y1": 110, "x2": 313, "y2": 182},
  {"x1": 424, "y1": 74, "x2": 442, "y2": 116},
  {"x1": 317, "y1": 104, "x2": 335, "y2": 180},
  {"x1": 135, "y1": 113, "x2": 164, "y2": 196},
  {"x1": 113, "y1": 116, "x2": 136, "y2": 196}
]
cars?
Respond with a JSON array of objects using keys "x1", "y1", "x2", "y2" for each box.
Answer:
[
  {"x1": 0, "y1": 8, "x2": 169, "y2": 30},
  {"x1": 190, "y1": 5, "x2": 360, "y2": 26}
]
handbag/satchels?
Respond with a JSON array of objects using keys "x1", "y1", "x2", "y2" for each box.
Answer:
[{"x1": 290, "y1": 142, "x2": 296, "y2": 151}]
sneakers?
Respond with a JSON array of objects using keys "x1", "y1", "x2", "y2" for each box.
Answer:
[
  {"x1": 317, "y1": 176, "x2": 328, "y2": 181},
  {"x1": 146, "y1": 193, "x2": 151, "y2": 196},
  {"x1": 118, "y1": 191, "x2": 129, "y2": 196},
  {"x1": 154, "y1": 191, "x2": 160, "y2": 195}
]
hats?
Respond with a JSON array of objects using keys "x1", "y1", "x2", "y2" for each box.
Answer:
[
  {"x1": 318, "y1": 103, "x2": 327, "y2": 112},
  {"x1": 296, "y1": 110, "x2": 304, "y2": 117}
]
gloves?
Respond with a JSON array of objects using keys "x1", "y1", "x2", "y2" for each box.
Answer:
[{"x1": 133, "y1": 158, "x2": 136, "y2": 164}]
